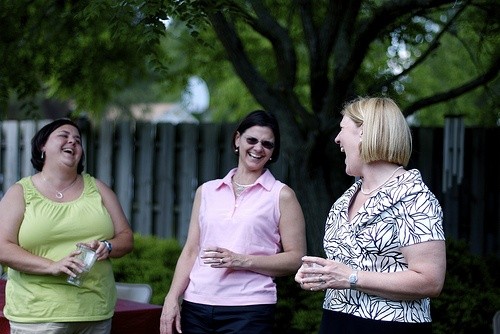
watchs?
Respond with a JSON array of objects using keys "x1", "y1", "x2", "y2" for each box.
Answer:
[
  {"x1": 349, "y1": 269, "x2": 359, "y2": 290},
  {"x1": 100, "y1": 240, "x2": 112, "y2": 254}
]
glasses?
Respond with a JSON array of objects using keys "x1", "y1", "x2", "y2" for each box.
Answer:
[{"x1": 240, "y1": 135, "x2": 274, "y2": 149}]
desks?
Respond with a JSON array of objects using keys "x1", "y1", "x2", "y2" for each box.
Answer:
[{"x1": 0, "y1": 280, "x2": 163, "y2": 334}]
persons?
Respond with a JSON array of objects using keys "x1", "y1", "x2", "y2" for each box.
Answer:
[
  {"x1": 295, "y1": 96, "x2": 446, "y2": 334},
  {"x1": 0, "y1": 119, "x2": 134, "y2": 334},
  {"x1": 160, "y1": 110, "x2": 307, "y2": 334}
]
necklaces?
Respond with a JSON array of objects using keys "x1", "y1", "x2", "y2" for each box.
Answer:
[
  {"x1": 361, "y1": 166, "x2": 404, "y2": 195},
  {"x1": 41, "y1": 172, "x2": 76, "y2": 199},
  {"x1": 232, "y1": 180, "x2": 254, "y2": 196}
]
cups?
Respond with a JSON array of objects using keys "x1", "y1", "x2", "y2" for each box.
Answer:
[
  {"x1": 301, "y1": 256, "x2": 326, "y2": 292},
  {"x1": 65, "y1": 243, "x2": 100, "y2": 289}
]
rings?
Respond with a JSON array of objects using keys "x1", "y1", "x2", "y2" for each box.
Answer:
[
  {"x1": 220, "y1": 259, "x2": 223, "y2": 263},
  {"x1": 319, "y1": 277, "x2": 322, "y2": 282}
]
